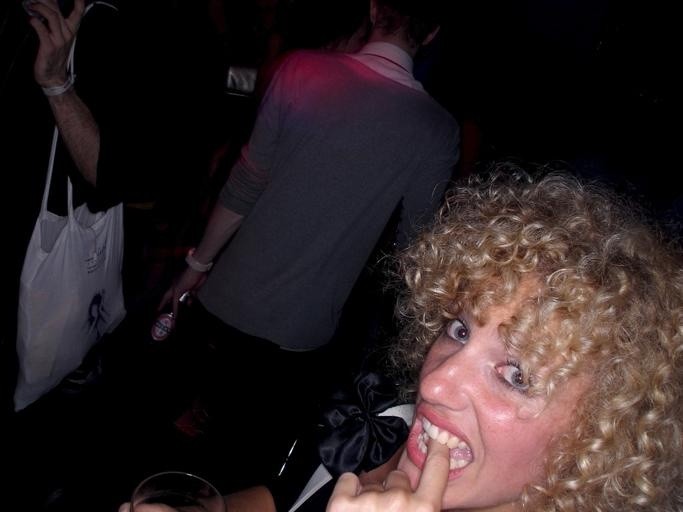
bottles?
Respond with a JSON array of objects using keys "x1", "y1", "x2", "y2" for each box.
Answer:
[{"x1": 144, "y1": 288, "x2": 192, "y2": 353}]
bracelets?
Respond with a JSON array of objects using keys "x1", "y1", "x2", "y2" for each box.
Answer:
[{"x1": 184, "y1": 248, "x2": 213, "y2": 274}]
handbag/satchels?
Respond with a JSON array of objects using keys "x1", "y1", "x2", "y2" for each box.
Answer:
[{"x1": 13, "y1": 202, "x2": 127, "y2": 412}]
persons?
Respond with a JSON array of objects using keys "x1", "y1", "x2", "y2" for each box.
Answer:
[
  {"x1": 112, "y1": 2, "x2": 466, "y2": 475},
  {"x1": 1, "y1": 0, "x2": 151, "y2": 512},
  {"x1": 112, "y1": 158, "x2": 681, "y2": 511}
]
